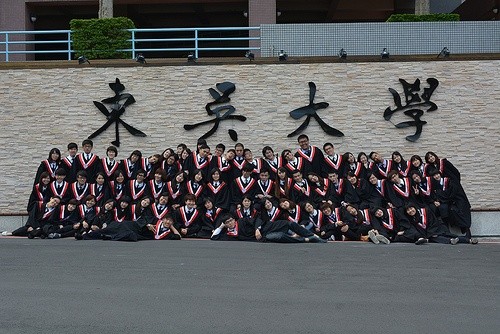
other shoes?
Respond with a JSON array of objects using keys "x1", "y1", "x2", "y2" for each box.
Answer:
[
  {"x1": 2, "y1": 231, "x2": 8, "y2": 236},
  {"x1": 471, "y1": 239, "x2": 479, "y2": 244},
  {"x1": 368, "y1": 231, "x2": 390, "y2": 244},
  {"x1": 27, "y1": 229, "x2": 48, "y2": 239},
  {"x1": 47, "y1": 233, "x2": 59, "y2": 239},
  {"x1": 450, "y1": 237, "x2": 459, "y2": 244},
  {"x1": 342, "y1": 235, "x2": 346, "y2": 241},
  {"x1": 331, "y1": 234, "x2": 335, "y2": 240},
  {"x1": 308, "y1": 236, "x2": 327, "y2": 243},
  {"x1": 74, "y1": 233, "x2": 111, "y2": 240}
]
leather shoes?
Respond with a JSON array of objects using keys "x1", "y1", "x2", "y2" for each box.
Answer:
[{"x1": 415, "y1": 238, "x2": 429, "y2": 245}]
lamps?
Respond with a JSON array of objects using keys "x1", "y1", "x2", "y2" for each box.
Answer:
[
  {"x1": 278, "y1": 50, "x2": 288, "y2": 61},
  {"x1": 380, "y1": 48, "x2": 389, "y2": 58},
  {"x1": 276, "y1": 8, "x2": 282, "y2": 16},
  {"x1": 339, "y1": 48, "x2": 347, "y2": 59},
  {"x1": 31, "y1": 17, "x2": 36, "y2": 21},
  {"x1": 136, "y1": 54, "x2": 147, "y2": 64},
  {"x1": 245, "y1": 53, "x2": 255, "y2": 61},
  {"x1": 243, "y1": 9, "x2": 248, "y2": 17},
  {"x1": 187, "y1": 55, "x2": 195, "y2": 65},
  {"x1": 493, "y1": 7, "x2": 498, "y2": 13},
  {"x1": 78, "y1": 56, "x2": 90, "y2": 65},
  {"x1": 437, "y1": 47, "x2": 450, "y2": 57}
]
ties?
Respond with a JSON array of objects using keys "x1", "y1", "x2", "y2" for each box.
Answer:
[{"x1": 44, "y1": 150, "x2": 427, "y2": 232}]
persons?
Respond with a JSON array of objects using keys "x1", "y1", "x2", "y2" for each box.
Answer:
[
  {"x1": 229, "y1": 164, "x2": 258, "y2": 204},
  {"x1": 295, "y1": 133, "x2": 326, "y2": 178},
  {"x1": 32, "y1": 138, "x2": 162, "y2": 180},
  {"x1": 280, "y1": 149, "x2": 316, "y2": 179},
  {"x1": 2, "y1": 195, "x2": 60, "y2": 239},
  {"x1": 26, "y1": 170, "x2": 154, "y2": 214},
  {"x1": 320, "y1": 202, "x2": 479, "y2": 244},
  {"x1": 44, "y1": 191, "x2": 230, "y2": 239},
  {"x1": 428, "y1": 168, "x2": 472, "y2": 239},
  {"x1": 255, "y1": 167, "x2": 359, "y2": 205},
  {"x1": 210, "y1": 213, "x2": 328, "y2": 243},
  {"x1": 233, "y1": 196, "x2": 322, "y2": 235},
  {"x1": 342, "y1": 150, "x2": 461, "y2": 206},
  {"x1": 149, "y1": 167, "x2": 230, "y2": 208},
  {"x1": 262, "y1": 145, "x2": 288, "y2": 180},
  {"x1": 162, "y1": 139, "x2": 263, "y2": 179},
  {"x1": 74, "y1": 213, "x2": 182, "y2": 241},
  {"x1": 322, "y1": 142, "x2": 345, "y2": 178}
]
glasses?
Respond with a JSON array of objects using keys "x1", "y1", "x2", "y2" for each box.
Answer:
[
  {"x1": 53, "y1": 198, "x2": 59, "y2": 204},
  {"x1": 84, "y1": 145, "x2": 92, "y2": 148},
  {"x1": 225, "y1": 218, "x2": 233, "y2": 224},
  {"x1": 79, "y1": 176, "x2": 86, "y2": 179},
  {"x1": 299, "y1": 140, "x2": 308, "y2": 143},
  {"x1": 285, "y1": 152, "x2": 289, "y2": 157},
  {"x1": 138, "y1": 174, "x2": 144, "y2": 176}
]
